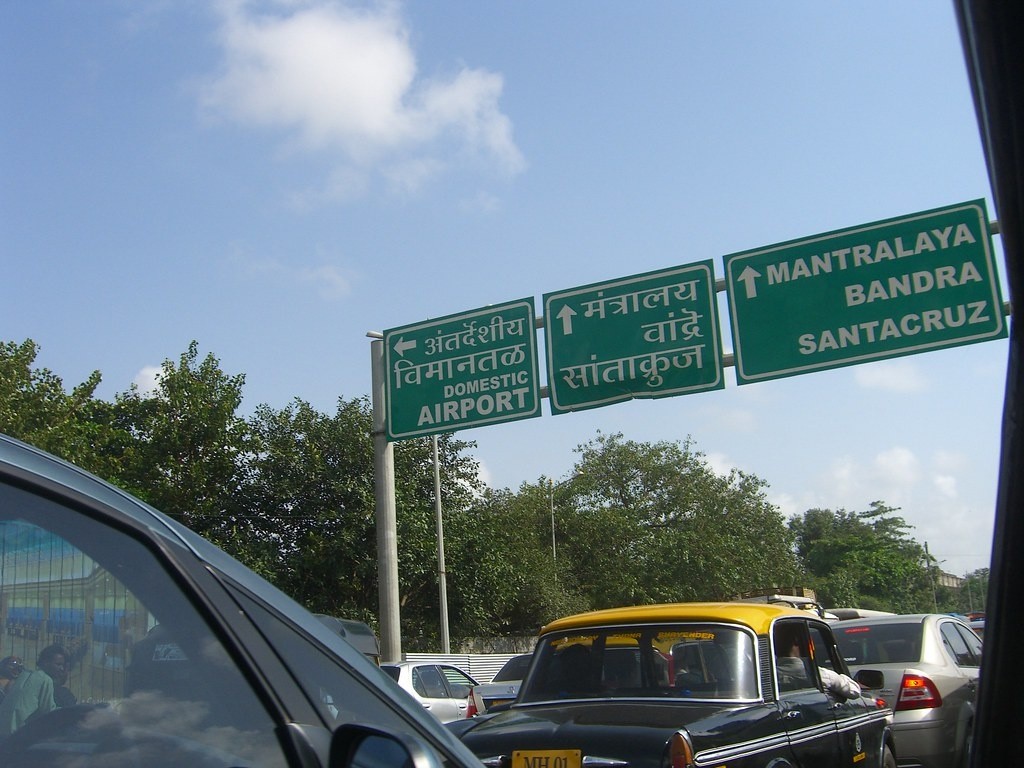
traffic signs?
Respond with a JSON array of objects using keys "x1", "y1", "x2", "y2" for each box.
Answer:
[
  {"x1": 541, "y1": 258, "x2": 725, "y2": 415},
  {"x1": 385, "y1": 296, "x2": 541, "y2": 442},
  {"x1": 722, "y1": 199, "x2": 1009, "y2": 387}
]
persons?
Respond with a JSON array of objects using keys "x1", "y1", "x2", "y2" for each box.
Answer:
[
  {"x1": 774, "y1": 625, "x2": 862, "y2": 699},
  {"x1": 0, "y1": 645, "x2": 76, "y2": 741}
]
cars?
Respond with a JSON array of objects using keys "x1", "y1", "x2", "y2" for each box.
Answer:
[
  {"x1": 827, "y1": 613, "x2": 984, "y2": 768},
  {"x1": 724, "y1": 593, "x2": 984, "y2": 645},
  {"x1": 466, "y1": 653, "x2": 556, "y2": 720},
  {"x1": 429, "y1": 593, "x2": 899, "y2": 768},
  {"x1": 379, "y1": 660, "x2": 482, "y2": 725}
]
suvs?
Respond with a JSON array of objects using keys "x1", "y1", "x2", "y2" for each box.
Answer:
[{"x1": 0, "y1": 430, "x2": 489, "y2": 768}]
